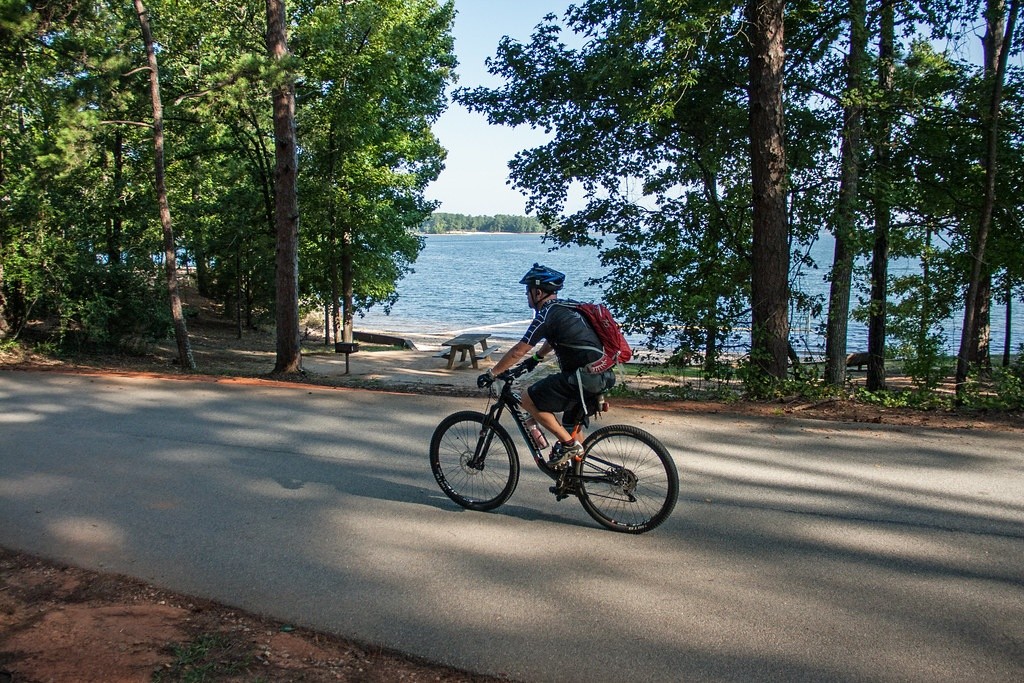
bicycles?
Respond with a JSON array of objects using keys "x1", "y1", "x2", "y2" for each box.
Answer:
[{"x1": 426, "y1": 365, "x2": 680, "y2": 534}]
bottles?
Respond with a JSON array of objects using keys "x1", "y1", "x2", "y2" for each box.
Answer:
[{"x1": 522, "y1": 412, "x2": 550, "y2": 450}]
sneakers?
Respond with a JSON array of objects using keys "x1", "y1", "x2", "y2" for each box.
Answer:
[
  {"x1": 549, "y1": 475, "x2": 577, "y2": 494},
  {"x1": 547, "y1": 439, "x2": 585, "y2": 466}
]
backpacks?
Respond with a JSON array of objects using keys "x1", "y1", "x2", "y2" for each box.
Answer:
[{"x1": 543, "y1": 299, "x2": 633, "y2": 374}]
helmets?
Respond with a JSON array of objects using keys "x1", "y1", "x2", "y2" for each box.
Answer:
[{"x1": 518, "y1": 262, "x2": 566, "y2": 289}]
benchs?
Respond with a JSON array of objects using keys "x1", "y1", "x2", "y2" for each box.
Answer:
[
  {"x1": 846, "y1": 353, "x2": 869, "y2": 372},
  {"x1": 431, "y1": 347, "x2": 450, "y2": 359},
  {"x1": 474, "y1": 344, "x2": 501, "y2": 359}
]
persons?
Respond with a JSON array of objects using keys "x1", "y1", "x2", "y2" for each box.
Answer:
[{"x1": 477, "y1": 262, "x2": 616, "y2": 467}]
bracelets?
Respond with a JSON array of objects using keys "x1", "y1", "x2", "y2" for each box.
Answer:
[{"x1": 533, "y1": 351, "x2": 544, "y2": 362}]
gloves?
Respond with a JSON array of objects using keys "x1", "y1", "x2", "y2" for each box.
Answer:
[
  {"x1": 516, "y1": 351, "x2": 544, "y2": 373},
  {"x1": 477, "y1": 368, "x2": 497, "y2": 389}
]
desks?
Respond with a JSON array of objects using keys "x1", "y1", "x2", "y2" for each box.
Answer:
[{"x1": 442, "y1": 334, "x2": 491, "y2": 369}]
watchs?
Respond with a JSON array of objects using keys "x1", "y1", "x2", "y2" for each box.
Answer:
[{"x1": 488, "y1": 368, "x2": 497, "y2": 378}]
